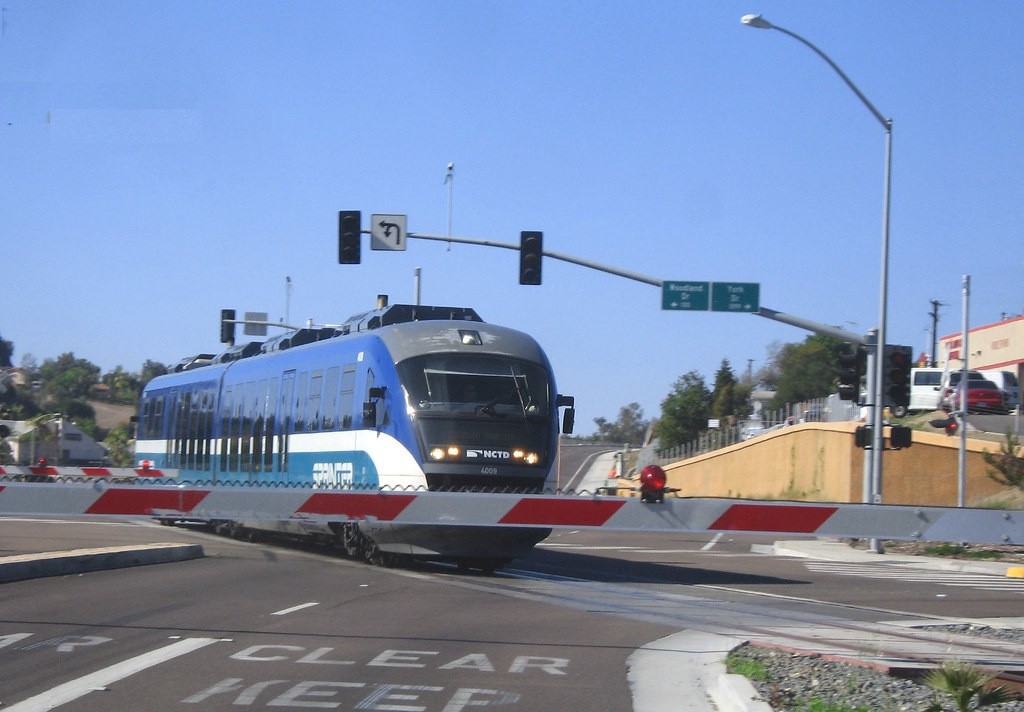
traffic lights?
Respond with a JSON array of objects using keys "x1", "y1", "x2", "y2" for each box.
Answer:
[
  {"x1": 339, "y1": 209, "x2": 362, "y2": 265},
  {"x1": 836, "y1": 345, "x2": 863, "y2": 402},
  {"x1": 855, "y1": 425, "x2": 872, "y2": 448},
  {"x1": 890, "y1": 424, "x2": 912, "y2": 448},
  {"x1": 519, "y1": 230, "x2": 543, "y2": 286},
  {"x1": 882, "y1": 344, "x2": 913, "y2": 407}
]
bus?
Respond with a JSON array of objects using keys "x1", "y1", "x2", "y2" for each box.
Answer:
[{"x1": 125, "y1": 294, "x2": 564, "y2": 576}]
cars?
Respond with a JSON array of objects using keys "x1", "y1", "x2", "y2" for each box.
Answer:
[{"x1": 888, "y1": 368, "x2": 1020, "y2": 418}]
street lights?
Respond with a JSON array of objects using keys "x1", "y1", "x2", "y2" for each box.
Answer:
[
  {"x1": 30, "y1": 413, "x2": 63, "y2": 466},
  {"x1": 740, "y1": 13, "x2": 894, "y2": 555}
]
